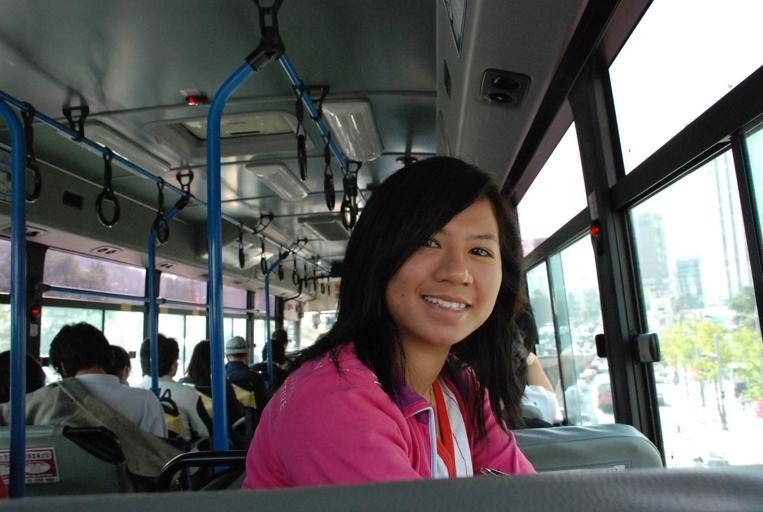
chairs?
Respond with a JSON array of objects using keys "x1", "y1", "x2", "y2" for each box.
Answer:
[
  {"x1": 512, "y1": 423, "x2": 665, "y2": 472},
  {"x1": 1, "y1": 427, "x2": 135, "y2": 495}
]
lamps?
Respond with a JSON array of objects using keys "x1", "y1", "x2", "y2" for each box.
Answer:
[
  {"x1": 321, "y1": 100, "x2": 382, "y2": 163},
  {"x1": 245, "y1": 163, "x2": 310, "y2": 203}
]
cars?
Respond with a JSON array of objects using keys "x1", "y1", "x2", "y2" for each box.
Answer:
[{"x1": 535, "y1": 318, "x2": 732, "y2": 465}]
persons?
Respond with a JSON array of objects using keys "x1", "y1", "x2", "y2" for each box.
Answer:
[
  {"x1": 240, "y1": 156, "x2": 536, "y2": 491},
  {"x1": 1, "y1": 297, "x2": 564, "y2": 497}
]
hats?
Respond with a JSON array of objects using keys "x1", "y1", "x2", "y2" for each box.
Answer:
[
  {"x1": 272, "y1": 329, "x2": 291, "y2": 343},
  {"x1": 226, "y1": 336, "x2": 248, "y2": 355}
]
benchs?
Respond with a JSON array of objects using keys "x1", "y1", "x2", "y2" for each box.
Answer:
[{"x1": 1, "y1": 463, "x2": 763, "y2": 511}]
